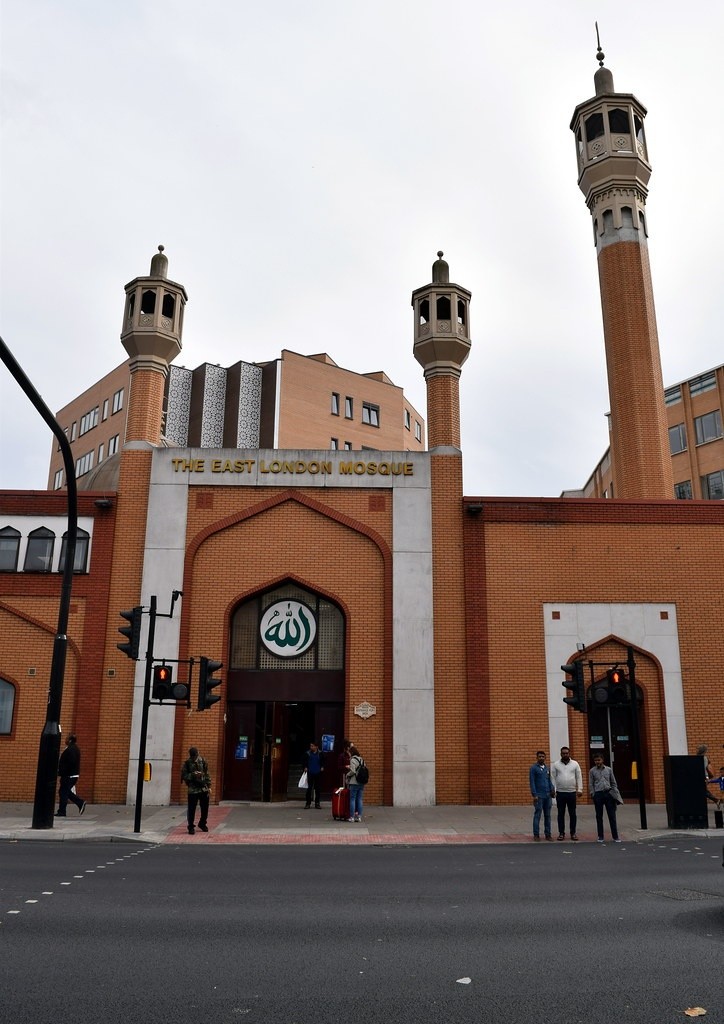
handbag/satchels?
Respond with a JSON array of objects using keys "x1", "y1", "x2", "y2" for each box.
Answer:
[
  {"x1": 298, "y1": 771, "x2": 308, "y2": 788},
  {"x1": 706, "y1": 762, "x2": 715, "y2": 780},
  {"x1": 71, "y1": 786, "x2": 76, "y2": 795}
]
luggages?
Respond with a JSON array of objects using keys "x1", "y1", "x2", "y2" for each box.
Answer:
[{"x1": 332, "y1": 786, "x2": 358, "y2": 821}]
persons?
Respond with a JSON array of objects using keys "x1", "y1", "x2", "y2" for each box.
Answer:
[
  {"x1": 338, "y1": 742, "x2": 366, "y2": 823},
  {"x1": 550, "y1": 747, "x2": 583, "y2": 841},
  {"x1": 708, "y1": 767, "x2": 724, "y2": 800},
  {"x1": 530, "y1": 751, "x2": 555, "y2": 841},
  {"x1": 302, "y1": 742, "x2": 325, "y2": 809},
  {"x1": 54, "y1": 732, "x2": 86, "y2": 816},
  {"x1": 589, "y1": 753, "x2": 624, "y2": 842},
  {"x1": 182, "y1": 747, "x2": 212, "y2": 834},
  {"x1": 696, "y1": 744, "x2": 721, "y2": 809}
]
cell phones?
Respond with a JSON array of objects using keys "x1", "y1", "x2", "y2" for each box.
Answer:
[{"x1": 197, "y1": 771, "x2": 202, "y2": 774}]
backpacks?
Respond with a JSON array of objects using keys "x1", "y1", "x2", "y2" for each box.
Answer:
[{"x1": 352, "y1": 757, "x2": 369, "y2": 785}]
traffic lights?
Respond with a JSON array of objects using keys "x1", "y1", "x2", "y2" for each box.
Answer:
[
  {"x1": 607, "y1": 669, "x2": 628, "y2": 703},
  {"x1": 198, "y1": 655, "x2": 224, "y2": 711},
  {"x1": 116, "y1": 604, "x2": 142, "y2": 659},
  {"x1": 152, "y1": 666, "x2": 172, "y2": 698},
  {"x1": 561, "y1": 660, "x2": 586, "y2": 713}
]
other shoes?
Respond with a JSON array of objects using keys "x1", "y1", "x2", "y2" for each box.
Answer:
[
  {"x1": 347, "y1": 817, "x2": 355, "y2": 823},
  {"x1": 198, "y1": 823, "x2": 208, "y2": 833},
  {"x1": 597, "y1": 838, "x2": 606, "y2": 843},
  {"x1": 557, "y1": 834, "x2": 565, "y2": 841},
  {"x1": 79, "y1": 801, "x2": 87, "y2": 816},
  {"x1": 303, "y1": 804, "x2": 311, "y2": 810},
  {"x1": 613, "y1": 838, "x2": 622, "y2": 843},
  {"x1": 717, "y1": 799, "x2": 721, "y2": 809},
  {"x1": 355, "y1": 817, "x2": 361, "y2": 822},
  {"x1": 545, "y1": 835, "x2": 554, "y2": 842},
  {"x1": 55, "y1": 813, "x2": 66, "y2": 817},
  {"x1": 315, "y1": 803, "x2": 322, "y2": 810},
  {"x1": 571, "y1": 835, "x2": 578, "y2": 841},
  {"x1": 189, "y1": 829, "x2": 195, "y2": 835},
  {"x1": 533, "y1": 836, "x2": 541, "y2": 842}
]
